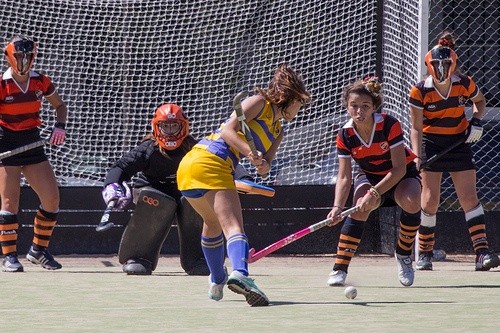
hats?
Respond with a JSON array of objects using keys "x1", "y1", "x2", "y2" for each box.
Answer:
[
  {"x1": 425, "y1": 44, "x2": 459, "y2": 84},
  {"x1": 4, "y1": 35, "x2": 36, "y2": 76}
]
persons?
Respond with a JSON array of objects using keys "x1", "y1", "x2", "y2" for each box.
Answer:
[
  {"x1": 327, "y1": 76, "x2": 423, "y2": 287},
  {"x1": 0, "y1": 33, "x2": 67, "y2": 270},
  {"x1": 407, "y1": 33, "x2": 500, "y2": 273},
  {"x1": 102, "y1": 104, "x2": 212, "y2": 275},
  {"x1": 176, "y1": 67, "x2": 310, "y2": 305}
]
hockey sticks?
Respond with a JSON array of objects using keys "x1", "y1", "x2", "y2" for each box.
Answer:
[
  {"x1": 96, "y1": 198, "x2": 117, "y2": 233},
  {"x1": 416, "y1": 137, "x2": 468, "y2": 172},
  {"x1": 248, "y1": 204, "x2": 361, "y2": 264},
  {"x1": 233, "y1": 89, "x2": 272, "y2": 182},
  {"x1": 0, "y1": 139, "x2": 51, "y2": 161}
]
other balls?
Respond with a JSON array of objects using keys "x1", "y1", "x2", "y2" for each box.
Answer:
[{"x1": 343, "y1": 285, "x2": 358, "y2": 299}]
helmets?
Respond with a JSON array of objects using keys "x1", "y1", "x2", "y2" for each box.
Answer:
[{"x1": 151, "y1": 104, "x2": 189, "y2": 151}]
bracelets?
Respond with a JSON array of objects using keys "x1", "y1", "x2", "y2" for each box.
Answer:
[
  {"x1": 55, "y1": 122, "x2": 65, "y2": 129},
  {"x1": 334, "y1": 206, "x2": 343, "y2": 211},
  {"x1": 367, "y1": 187, "x2": 381, "y2": 199}
]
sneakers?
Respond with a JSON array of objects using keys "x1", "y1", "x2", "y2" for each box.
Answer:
[
  {"x1": 394, "y1": 249, "x2": 414, "y2": 286},
  {"x1": 226, "y1": 269, "x2": 270, "y2": 306},
  {"x1": 475, "y1": 250, "x2": 500, "y2": 271},
  {"x1": 1, "y1": 251, "x2": 24, "y2": 272},
  {"x1": 25, "y1": 245, "x2": 62, "y2": 270},
  {"x1": 207, "y1": 266, "x2": 229, "y2": 301},
  {"x1": 416, "y1": 252, "x2": 432, "y2": 270},
  {"x1": 328, "y1": 270, "x2": 347, "y2": 287}
]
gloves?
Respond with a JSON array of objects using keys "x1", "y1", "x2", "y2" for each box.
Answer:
[
  {"x1": 465, "y1": 118, "x2": 484, "y2": 144},
  {"x1": 48, "y1": 122, "x2": 65, "y2": 145}
]
